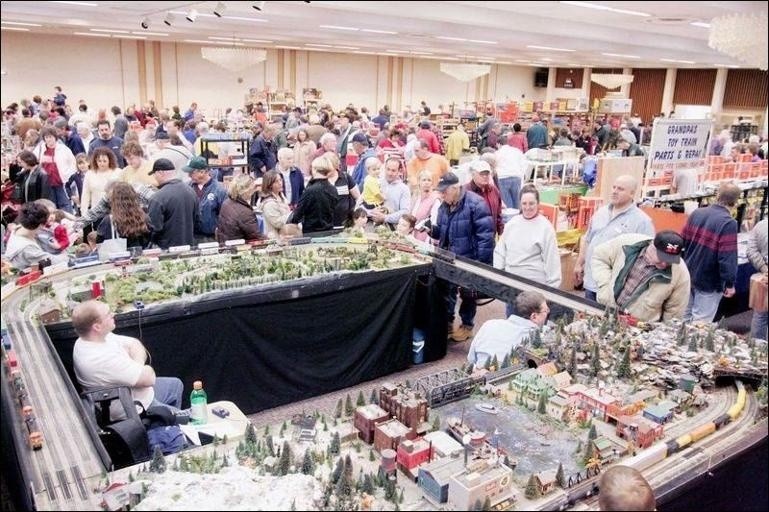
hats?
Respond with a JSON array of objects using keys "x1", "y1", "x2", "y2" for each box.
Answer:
[
  {"x1": 655, "y1": 231, "x2": 684, "y2": 262},
  {"x1": 181, "y1": 158, "x2": 208, "y2": 172},
  {"x1": 144, "y1": 407, "x2": 190, "y2": 426},
  {"x1": 434, "y1": 173, "x2": 457, "y2": 192},
  {"x1": 470, "y1": 162, "x2": 492, "y2": 175},
  {"x1": 345, "y1": 133, "x2": 368, "y2": 143},
  {"x1": 148, "y1": 158, "x2": 175, "y2": 174}
]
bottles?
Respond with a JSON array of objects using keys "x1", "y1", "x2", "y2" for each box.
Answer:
[{"x1": 191, "y1": 379, "x2": 209, "y2": 426}]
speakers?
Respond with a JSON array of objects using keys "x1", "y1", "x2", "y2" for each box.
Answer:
[{"x1": 535, "y1": 71, "x2": 547, "y2": 88}]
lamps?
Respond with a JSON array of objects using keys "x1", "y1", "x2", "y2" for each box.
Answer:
[
  {"x1": 590, "y1": 68, "x2": 635, "y2": 91},
  {"x1": 705, "y1": 1, "x2": 768, "y2": 71},
  {"x1": 201, "y1": 31, "x2": 268, "y2": 75},
  {"x1": 439, "y1": 53, "x2": 491, "y2": 83},
  {"x1": 140, "y1": 1, "x2": 312, "y2": 30}
]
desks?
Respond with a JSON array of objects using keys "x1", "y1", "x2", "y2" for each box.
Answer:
[
  {"x1": 31, "y1": 239, "x2": 449, "y2": 414},
  {"x1": 200, "y1": 133, "x2": 251, "y2": 174},
  {"x1": 103, "y1": 340, "x2": 768, "y2": 512},
  {"x1": 182, "y1": 400, "x2": 259, "y2": 446}
]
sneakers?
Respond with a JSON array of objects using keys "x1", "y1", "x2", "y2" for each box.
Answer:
[{"x1": 450, "y1": 322, "x2": 474, "y2": 342}]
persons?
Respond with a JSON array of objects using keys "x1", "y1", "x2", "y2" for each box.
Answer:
[
  {"x1": 711, "y1": 115, "x2": 768, "y2": 206},
  {"x1": 599, "y1": 465, "x2": 657, "y2": 512},
  {"x1": 248, "y1": 102, "x2": 504, "y2": 341},
  {"x1": 467, "y1": 290, "x2": 550, "y2": 369},
  {"x1": 71, "y1": 299, "x2": 184, "y2": 413},
  {"x1": 475, "y1": 109, "x2": 676, "y2": 208},
  {"x1": 0, "y1": 87, "x2": 267, "y2": 277},
  {"x1": 491, "y1": 176, "x2": 768, "y2": 338}
]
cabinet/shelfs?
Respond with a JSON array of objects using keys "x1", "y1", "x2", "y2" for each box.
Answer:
[
  {"x1": 402, "y1": 112, "x2": 769, "y2": 323},
  {"x1": 243, "y1": 94, "x2": 322, "y2": 120}
]
